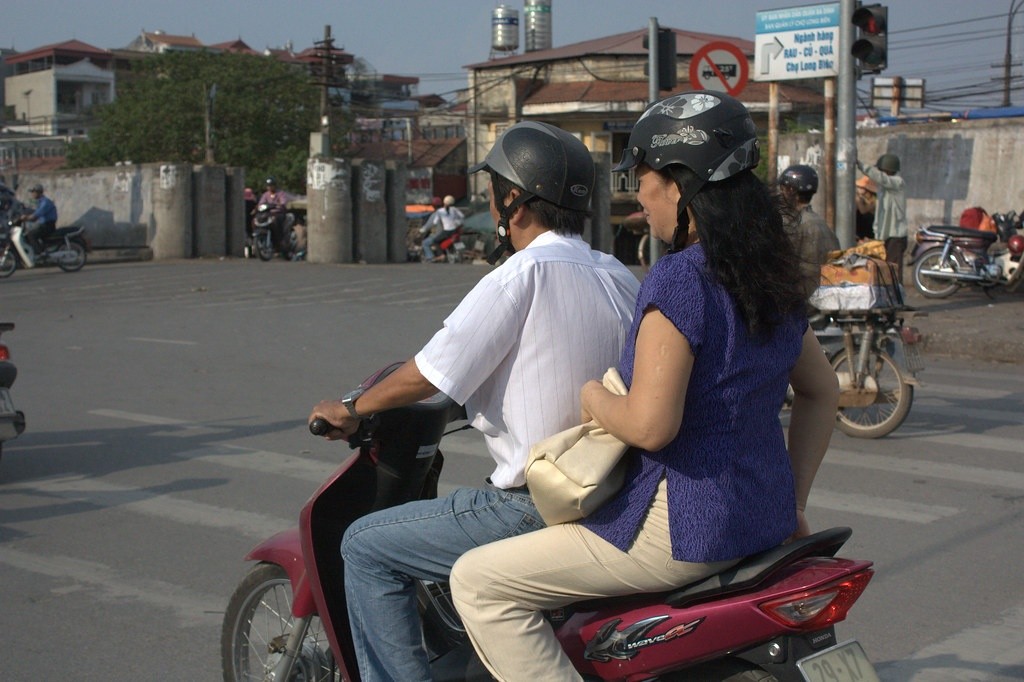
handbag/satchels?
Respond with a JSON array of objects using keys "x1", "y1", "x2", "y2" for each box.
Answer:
[{"x1": 521, "y1": 369, "x2": 639, "y2": 526}]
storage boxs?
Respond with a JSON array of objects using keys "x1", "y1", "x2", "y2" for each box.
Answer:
[{"x1": 820, "y1": 256, "x2": 898, "y2": 285}]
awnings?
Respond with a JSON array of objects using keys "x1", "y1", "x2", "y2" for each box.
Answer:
[{"x1": 404, "y1": 204, "x2": 435, "y2": 218}]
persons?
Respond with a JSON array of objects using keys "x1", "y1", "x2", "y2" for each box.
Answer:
[
  {"x1": 418, "y1": 195, "x2": 464, "y2": 262},
  {"x1": 290, "y1": 217, "x2": 307, "y2": 251},
  {"x1": 855, "y1": 147, "x2": 909, "y2": 287},
  {"x1": 14, "y1": 184, "x2": 56, "y2": 259},
  {"x1": 448, "y1": 91, "x2": 839, "y2": 682},
  {"x1": 251, "y1": 178, "x2": 288, "y2": 240},
  {"x1": 778, "y1": 166, "x2": 842, "y2": 300},
  {"x1": 309, "y1": 120, "x2": 640, "y2": 682}
]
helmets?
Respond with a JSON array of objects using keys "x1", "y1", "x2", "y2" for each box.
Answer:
[
  {"x1": 444, "y1": 194, "x2": 454, "y2": 206},
  {"x1": 462, "y1": 122, "x2": 596, "y2": 214},
  {"x1": 778, "y1": 166, "x2": 818, "y2": 196},
  {"x1": 27, "y1": 183, "x2": 43, "y2": 194},
  {"x1": 612, "y1": 93, "x2": 760, "y2": 187},
  {"x1": 264, "y1": 179, "x2": 272, "y2": 187},
  {"x1": 1008, "y1": 235, "x2": 1024, "y2": 256},
  {"x1": 876, "y1": 155, "x2": 900, "y2": 177},
  {"x1": 431, "y1": 196, "x2": 441, "y2": 209}
]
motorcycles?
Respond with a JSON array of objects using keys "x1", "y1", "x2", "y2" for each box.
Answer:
[
  {"x1": 247, "y1": 202, "x2": 295, "y2": 261},
  {"x1": 220, "y1": 360, "x2": 883, "y2": 682},
  {"x1": 0, "y1": 323, "x2": 26, "y2": 461},
  {"x1": 413, "y1": 223, "x2": 464, "y2": 265},
  {"x1": 906, "y1": 208, "x2": 1024, "y2": 302},
  {"x1": 0, "y1": 214, "x2": 93, "y2": 279},
  {"x1": 783, "y1": 308, "x2": 922, "y2": 439}
]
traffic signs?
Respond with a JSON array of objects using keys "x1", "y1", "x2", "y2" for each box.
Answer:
[{"x1": 753, "y1": 2, "x2": 840, "y2": 80}]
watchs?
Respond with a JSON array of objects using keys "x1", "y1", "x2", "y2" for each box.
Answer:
[{"x1": 341, "y1": 388, "x2": 375, "y2": 420}]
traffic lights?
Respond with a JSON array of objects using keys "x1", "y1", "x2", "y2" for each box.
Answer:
[
  {"x1": 641, "y1": 29, "x2": 677, "y2": 90},
  {"x1": 850, "y1": 5, "x2": 887, "y2": 71}
]
